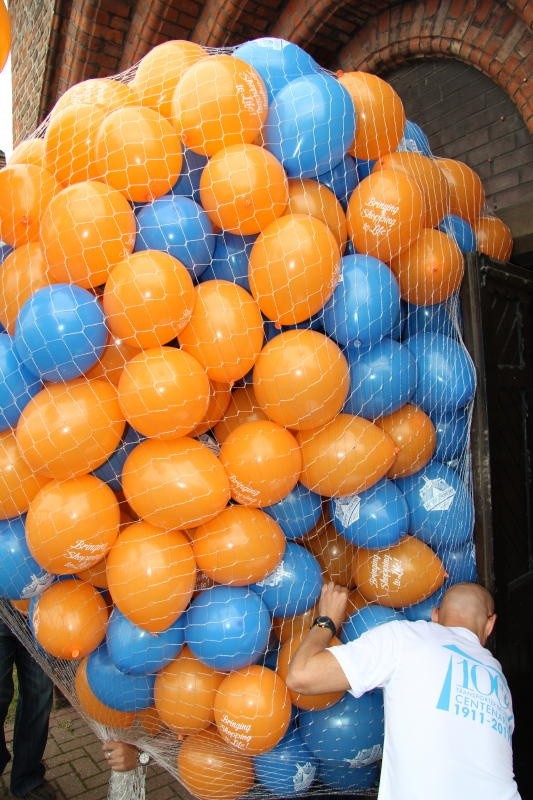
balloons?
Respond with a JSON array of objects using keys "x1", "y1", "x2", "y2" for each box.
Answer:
[
  {"x1": 470, "y1": 217, "x2": 513, "y2": 264},
  {"x1": 0, "y1": 0, "x2": 13, "y2": 75},
  {"x1": 1, "y1": 37, "x2": 483, "y2": 741}
]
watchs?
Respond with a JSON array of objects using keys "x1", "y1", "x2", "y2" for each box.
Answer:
[{"x1": 135, "y1": 748, "x2": 151, "y2": 766}]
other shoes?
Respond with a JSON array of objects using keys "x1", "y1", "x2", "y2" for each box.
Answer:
[
  {"x1": 0, "y1": 776, "x2": 10, "y2": 800},
  {"x1": 27, "y1": 777, "x2": 56, "y2": 799}
]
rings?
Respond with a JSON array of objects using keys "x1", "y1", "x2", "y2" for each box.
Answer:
[{"x1": 106, "y1": 751, "x2": 112, "y2": 758}]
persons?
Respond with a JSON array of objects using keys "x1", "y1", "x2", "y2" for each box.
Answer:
[
  {"x1": 0, "y1": 598, "x2": 63, "y2": 800},
  {"x1": 101, "y1": 730, "x2": 186, "y2": 772},
  {"x1": 285, "y1": 581, "x2": 524, "y2": 800}
]
